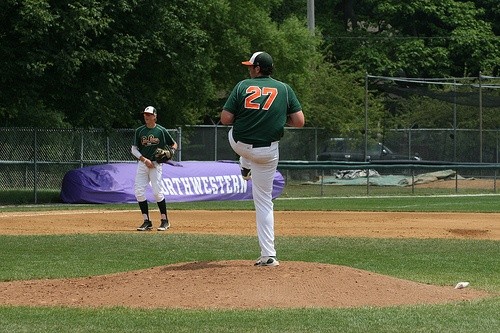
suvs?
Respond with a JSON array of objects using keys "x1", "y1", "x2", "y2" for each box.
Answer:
[{"x1": 317, "y1": 137, "x2": 423, "y2": 170}]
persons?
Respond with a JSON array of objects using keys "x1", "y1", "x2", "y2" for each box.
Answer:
[
  {"x1": 220, "y1": 51, "x2": 305, "y2": 267},
  {"x1": 131, "y1": 105, "x2": 178, "y2": 231}
]
